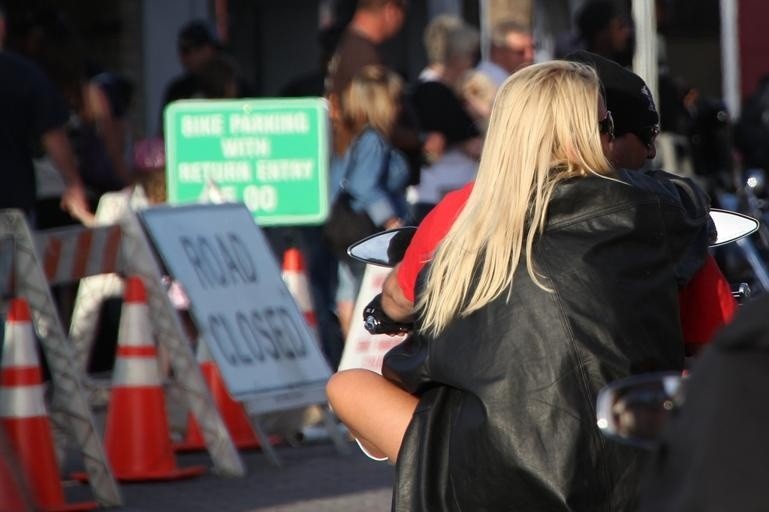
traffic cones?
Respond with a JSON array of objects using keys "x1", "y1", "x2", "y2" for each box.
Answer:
[
  {"x1": 170, "y1": 323, "x2": 281, "y2": 451},
  {"x1": 280, "y1": 249, "x2": 319, "y2": 343},
  {"x1": 1, "y1": 296, "x2": 103, "y2": 510},
  {"x1": 68, "y1": 277, "x2": 206, "y2": 480}
]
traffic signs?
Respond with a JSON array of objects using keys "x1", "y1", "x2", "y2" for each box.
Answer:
[{"x1": 163, "y1": 95, "x2": 330, "y2": 227}]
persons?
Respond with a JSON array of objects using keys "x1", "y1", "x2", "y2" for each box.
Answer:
[
  {"x1": 0, "y1": 2, "x2": 768, "y2": 364},
  {"x1": 325, "y1": 60, "x2": 716, "y2": 511}
]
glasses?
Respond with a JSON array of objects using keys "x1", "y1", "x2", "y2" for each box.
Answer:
[
  {"x1": 626, "y1": 125, "x2": 660, "y2": 150},
  {"x1": 597, "y1": 109, "x2": 617, "y2": 140}
]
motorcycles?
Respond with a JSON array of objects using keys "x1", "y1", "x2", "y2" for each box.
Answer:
[
  {"x1": 698, "y1": 164, "x2": 767, "y2": 260},
  {"x1": 343, "y1": 204, "x2": 757, "y2": 337}
]
